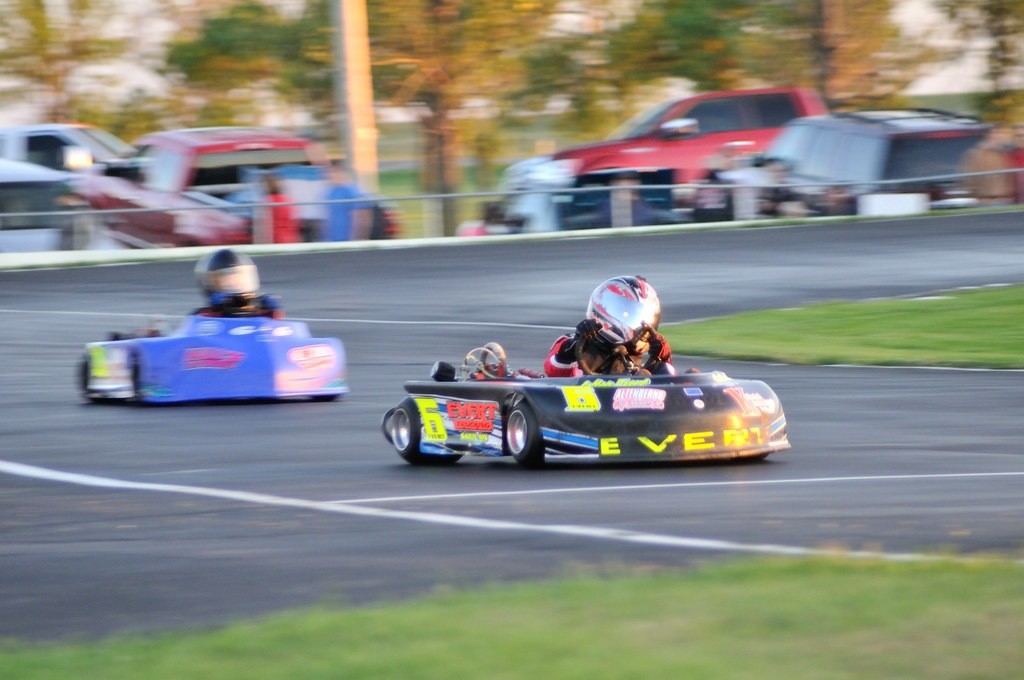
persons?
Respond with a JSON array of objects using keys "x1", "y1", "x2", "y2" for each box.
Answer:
[
  {"x1": 544, "y1": 276, "x2": 699, "y2": 378},
  {"x1": 186, "y1": 248, "x2": 285, "y2": 320}
]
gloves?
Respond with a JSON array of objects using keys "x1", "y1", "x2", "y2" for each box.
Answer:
[
  {"x1": 574, "y1": 319, "x2": 603, "y2": 341},
  {"x1": 647, "y1": 334, "x2": 672, "y2": 363}
]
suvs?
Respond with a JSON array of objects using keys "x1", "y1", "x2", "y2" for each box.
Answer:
[{"x1": 694, "y1": 104, "x2": 1002, "y2": 232}]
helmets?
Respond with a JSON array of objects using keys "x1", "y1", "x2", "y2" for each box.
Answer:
[
  {"x1": 589, "y1": 276, "x2": 661, "y2": 352},
  {"x1": 196, "y1": 248, "x2": 261, "y2": 298}
]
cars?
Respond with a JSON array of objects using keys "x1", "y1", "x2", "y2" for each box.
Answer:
[
  {"x1": 2, "y1": 119, "x2": 145, "y2": 177},
  {"x1": 500, "y1": 83, "x2": 834, "y2": 236},
  {"x1": 80, "y1": 121, "x2": 404, "y2": 265},
  {"x1": 1, "y1": 157, "x2": 126, "y2": 258}
]
